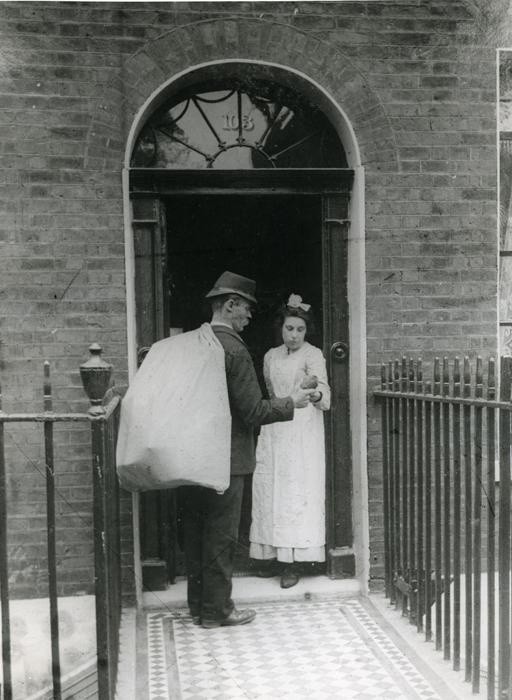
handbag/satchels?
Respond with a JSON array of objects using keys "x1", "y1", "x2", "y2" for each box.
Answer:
[{"x1": 112, "y1": 320, "x2": 236, "y2": 496}]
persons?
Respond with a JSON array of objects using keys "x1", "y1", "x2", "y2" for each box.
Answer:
[
  {"x1": 247, "y1": 290, "x2": 335, "y2": 588},
  {"x1": 171, "y1": 271, "x2": 314, "y2": 629}
]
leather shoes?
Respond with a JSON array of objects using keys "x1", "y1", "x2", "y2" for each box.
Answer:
[
  {"x1": 192, "y1": 615, "x2": 201, "y2": 626},
  {"x1": 202, "y1": 604, "x2": 256, "y2": 629},
  {"x1": 280, "y1": 571, "x2": 299, "y2": 589},
  {"x1": 256, "y1": 563, "x2": 276, "y2": 578}
]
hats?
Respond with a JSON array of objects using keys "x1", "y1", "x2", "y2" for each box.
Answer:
[{"x1": 204, "y1": 271, "x2": 259, "y2": 303}]
